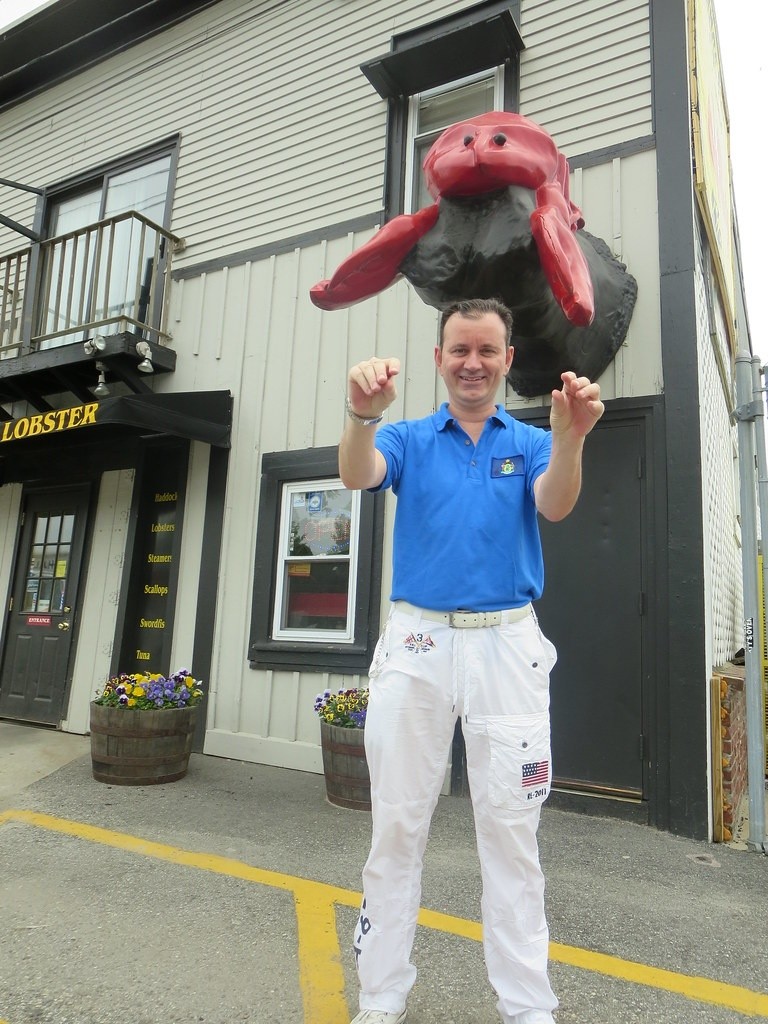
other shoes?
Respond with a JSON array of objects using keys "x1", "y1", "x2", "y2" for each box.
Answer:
[{"x1": 349, "y1": 1010, "x2": 408, "y2": 1024}]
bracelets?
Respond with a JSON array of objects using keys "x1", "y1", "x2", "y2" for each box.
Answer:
[{"x1": 347, "y1": 396, "x2": 384, "y2": 425}]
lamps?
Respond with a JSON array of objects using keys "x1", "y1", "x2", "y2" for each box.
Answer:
[
  {"x1": 135, "y1": 342, "x2": 154, "y2": 372},
  {"x1": 83, "y1": 334, "x2": 107, "y2": 354},
  {"x1": 93, "y1": 362, "x2": 110, "y2": 395}
]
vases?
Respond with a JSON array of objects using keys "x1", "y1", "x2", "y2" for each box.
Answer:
[
  {"x1": 92, "y1": 699, "x2": 196, "y2": 786},
  {"x1": 320, "y1": 719, "x2": 372, "y2": 811}
]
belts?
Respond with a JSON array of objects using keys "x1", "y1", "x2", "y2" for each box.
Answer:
[{"x1": 394, "y1": 600, "x2": 532, "y2": 628}]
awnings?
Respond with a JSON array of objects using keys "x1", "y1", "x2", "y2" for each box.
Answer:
[{"x1": 0, "y1": 390, "x2": 234, "y2": 486}]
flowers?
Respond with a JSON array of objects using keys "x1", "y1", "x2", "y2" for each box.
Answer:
[
  {"x1": 96, "y1": 671, "x2": 204, "y2": 710},
  {"x1": 315, "y1": 687, "x2": 371, "y2": 728}
]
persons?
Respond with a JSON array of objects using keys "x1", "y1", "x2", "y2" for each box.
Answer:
[{"x1": 338, "y1": 298, "x2": 606, "y2": 1024}]
